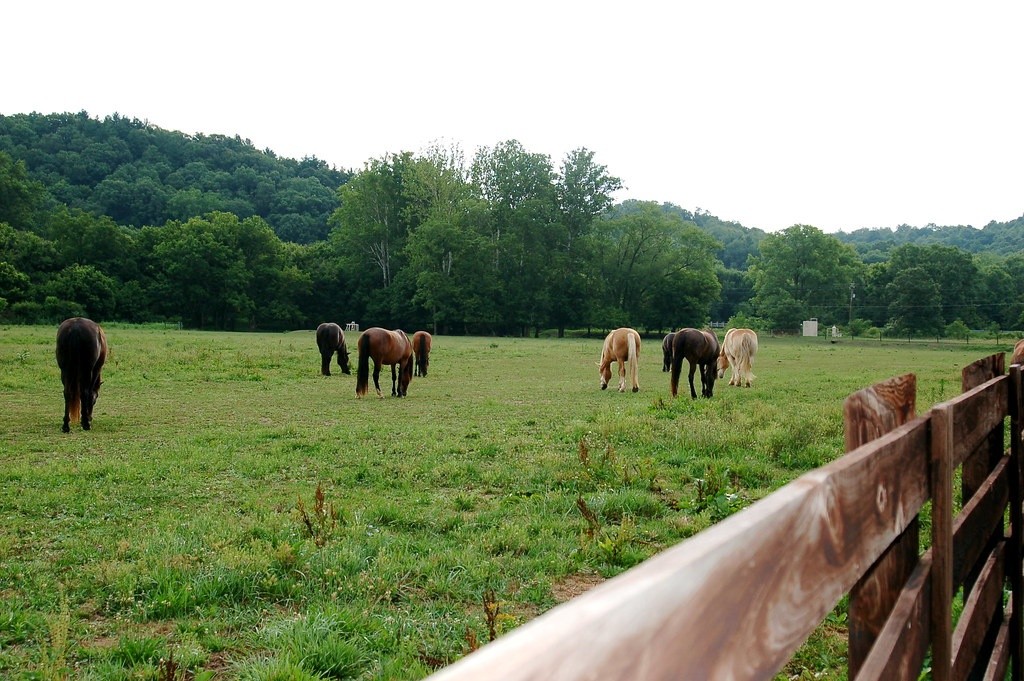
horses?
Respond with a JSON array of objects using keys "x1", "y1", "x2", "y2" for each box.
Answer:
[
  {"x1": 660, "y1": 328, "x2": 758, "y2": 399},
  {"x1": 594, "y1": 327, "x2": 641, "y2": 393},
  {"x1": 356, "y1": 326, "x2": 433, "y2": 399},
  {"x1": 315, "y1": 323, "x2": 351, "y2": 376},
  {"x1": 55, "y1": 317, "x2": 106, "y2": 434}
]
